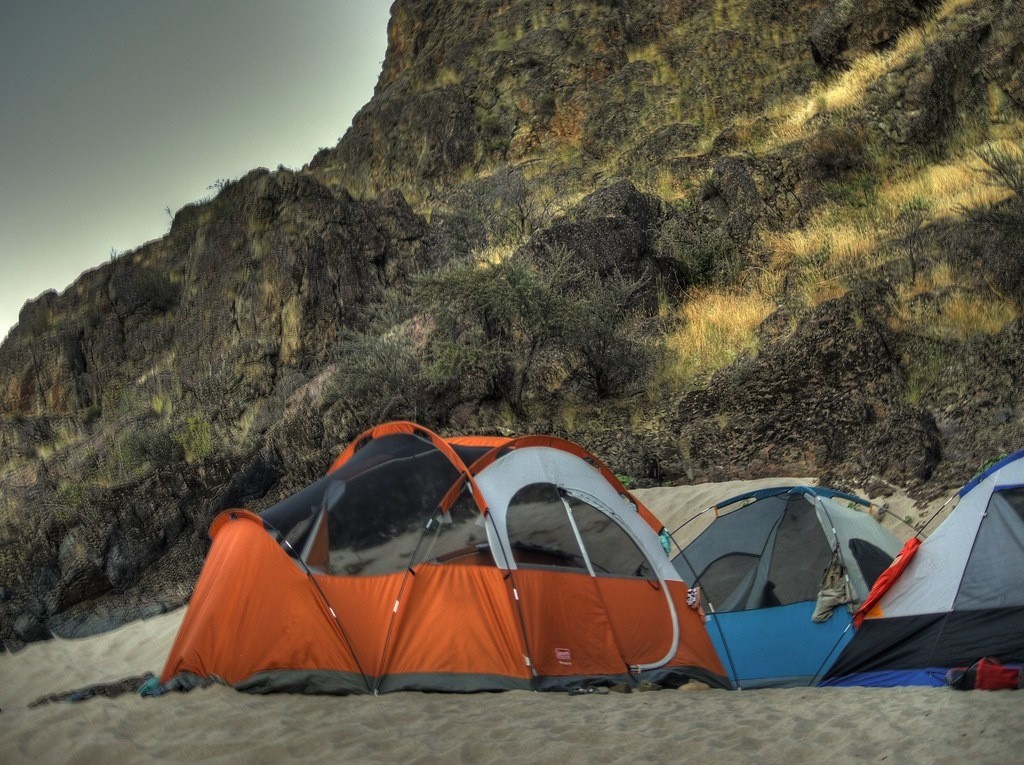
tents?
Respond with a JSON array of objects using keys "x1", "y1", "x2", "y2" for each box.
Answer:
[
  {"x1": 671, "y1": 484, "x2": 930, "y2": 688},
  {"x1": 798, "y1": 441, "x2": 1024, "y2": 688},
  {"x1": 162, "y1": 417, "x2": 740, "y2": 696}
]
